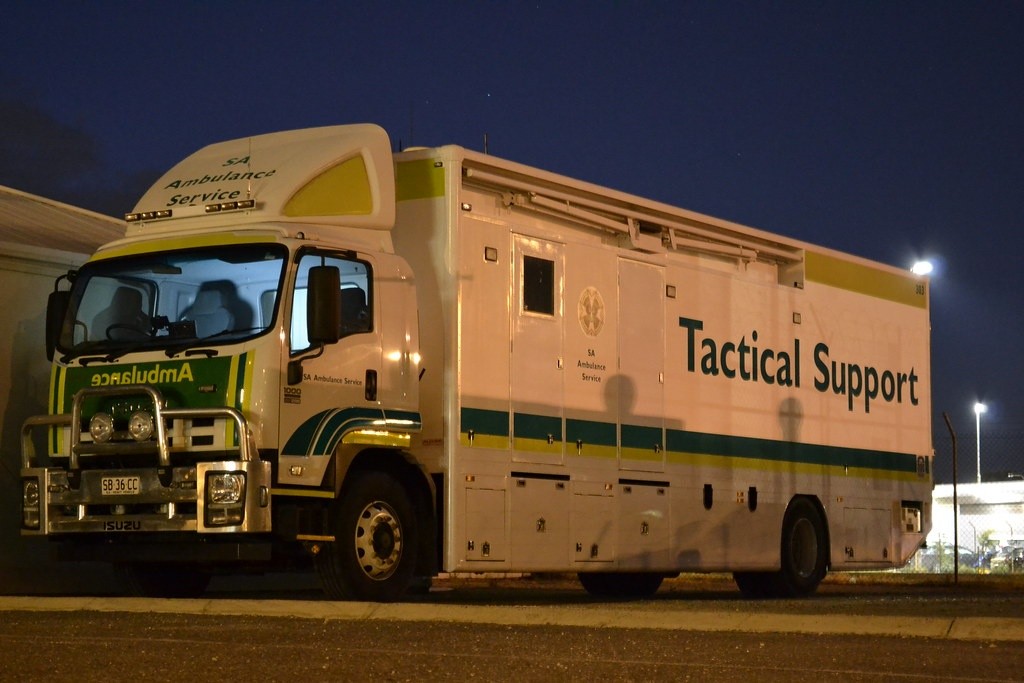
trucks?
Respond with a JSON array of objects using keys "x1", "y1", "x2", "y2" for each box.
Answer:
[{"x1": 18, "y1": 121, "x2": 936, "y2": 603}]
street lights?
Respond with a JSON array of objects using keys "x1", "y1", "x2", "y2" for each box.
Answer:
[{"x1": 971, "y1": 400, "x2": 989, "y2": 483}]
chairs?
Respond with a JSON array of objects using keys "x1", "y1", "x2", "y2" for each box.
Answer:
[
  {"x1": 91, "y1": 287, "x2": 148, "y2": 344},
  {"x1": 308, "y1": 281, "x2": 369, "y2": 347},
  {"x1": 178, "y1": 279, "x2": 254, "y2": 339}
]
